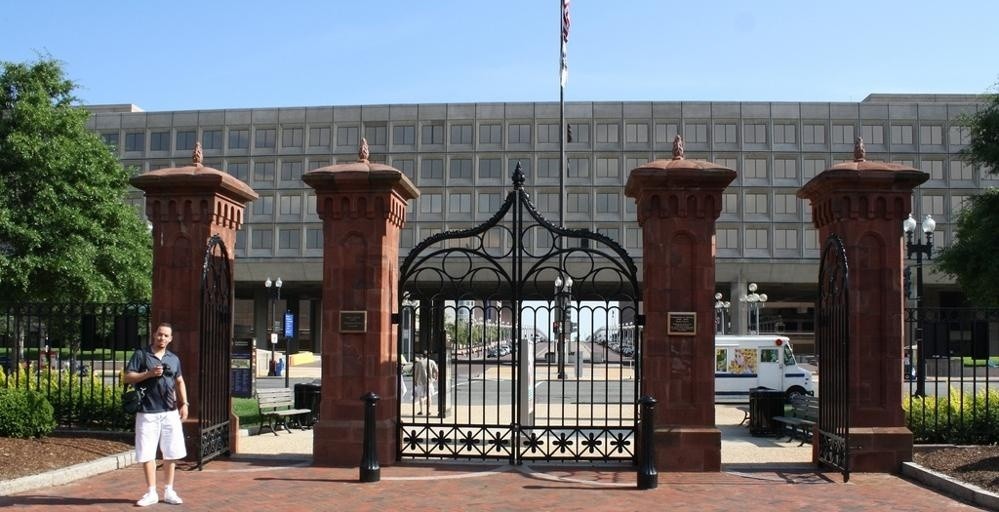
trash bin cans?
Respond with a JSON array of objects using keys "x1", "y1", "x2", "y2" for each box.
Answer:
[
  {"x1": 748, "y1": 385, "x2": 786, "y2": 439},
  {"x1": 293, "y1": 378, "x2": 321, "y2": 428}
]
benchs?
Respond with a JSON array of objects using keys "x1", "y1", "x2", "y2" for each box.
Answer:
[
  {"x1": 773, "y1": 395, "x2": 819, "y2": 447},
  {"x1": 256, "y1": 387, "x2": 312, "y2": 436}
]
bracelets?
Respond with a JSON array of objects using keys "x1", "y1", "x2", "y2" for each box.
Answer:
[{"x1": 179, "y1": 401, "x2": 191, "y2": 409}]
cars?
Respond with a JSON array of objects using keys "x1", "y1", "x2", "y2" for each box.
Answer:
[{"x1": 486, "y1": 334, "x2": 543, "y2": 356}]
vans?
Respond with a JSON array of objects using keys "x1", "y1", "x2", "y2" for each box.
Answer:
[{"x1": 713, "y1": 333, "x2": 814, "y2": 405}]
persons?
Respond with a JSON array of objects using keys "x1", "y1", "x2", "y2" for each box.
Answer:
[
  {"x1": 410, "y1": 356, "x2": 419, "y2": 397},
  {"x1": 121, "y1": 322, "x2": 189, "y2": 507},
  {"x1": 401, "y1": 354, "x2": 407, "y2": 401},
  {"x1": 414, "y1": 350, "x2": 439, "y2": 417}
]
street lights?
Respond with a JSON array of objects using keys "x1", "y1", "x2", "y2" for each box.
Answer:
[
  {"x1": 264, "y1": 276, "x2": 284, "y2": 375},
  {"x1": 609, "y1": 321, "x2": 636, "y2": 352},
  {"x1": 555, "y1": 274, "x2": 573, "y2": 379},
  {"x1": 904, "y1": 212, "x2": 938, "y2": 400},
  {"x1": 739, "y1": 282, "x2": 768, "y2": 335},
  {"x1": 714, "y1": 292, "x2": 732, "y2": 333}
]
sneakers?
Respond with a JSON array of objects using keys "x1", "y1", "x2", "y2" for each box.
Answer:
[
  {"x1": 135, "y1": 493, "x2": 159, "y2": 507},
  {"x1": 164, "y1": 491, "x2": 183, "y2": 505}
]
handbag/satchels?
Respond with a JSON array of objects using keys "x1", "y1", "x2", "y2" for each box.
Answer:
[{"x1": 121, "y1": 391, "x2": 142, "y2": 414}]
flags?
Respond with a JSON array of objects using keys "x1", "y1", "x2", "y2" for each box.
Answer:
[{"x1": 562, "y1": 0, "x2": 573, "y2": 88}]
